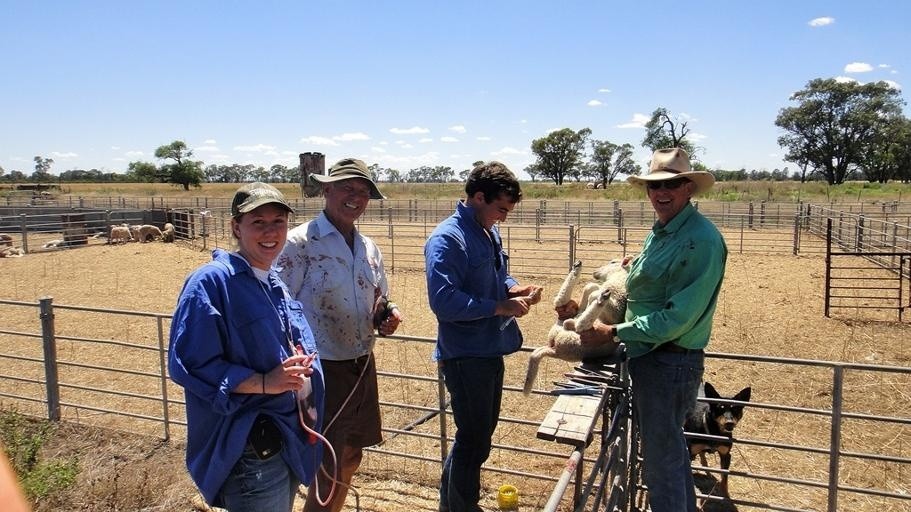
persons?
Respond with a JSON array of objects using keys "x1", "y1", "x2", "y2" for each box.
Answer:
[
  {"x1": 170, "y1": 182, "x2": 326, "y2": 512},
  {"x1": 554, "y1": 148, "x2": 729, "y2": 512},
  {"x1": 0, "y1": 446, "x2": 32, "y2": 510},
  {"x1": 269, "y1": 158, "x2": 402, "y2": 511},
  {"x1": 423, "y1": 162, "x2": 541, "y2": 512}
]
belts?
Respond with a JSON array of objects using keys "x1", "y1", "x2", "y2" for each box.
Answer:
[{"x1": 658, "y1": 343, "x2": 702, "y2": 353}]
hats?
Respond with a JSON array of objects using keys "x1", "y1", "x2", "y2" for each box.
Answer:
[
  {"x1": 626, "y1": 148, "x2": 715, "y2": 194},
  {"x1": 231, "y1": 183, "x2": 293, "y2": 219},
  {"x1": 309, "y1": 159, "x2": 384, "y2": 198}
]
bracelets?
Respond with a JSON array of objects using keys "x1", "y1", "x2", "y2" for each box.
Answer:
[{"x1": 263, "y1": 372, "x2": 265, "y2": 395}]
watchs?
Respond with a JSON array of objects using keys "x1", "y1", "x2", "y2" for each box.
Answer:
[{"x1": 612, "y1": 328, "x2": 620, "y2": 345}]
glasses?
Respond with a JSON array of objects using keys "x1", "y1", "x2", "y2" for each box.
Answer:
[{"x1": 647, "y1": 180, "x2": 688, "y2": 188}]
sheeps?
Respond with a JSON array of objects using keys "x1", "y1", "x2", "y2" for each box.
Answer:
[
  {"x1": 0, "y1": 233, "x2": 25, "y2": 258},
  {"x1": 42, "y1": 240, "x2": 66, "y2": 248},
  {"x1": 40, "y1": 191, "x2": 52, "y2": 200},
  {"x1": 107, "y1": 223, "x2": 176, "y2": 246},
  {"x1": 522, "y1": 254, "x2": 636, "y2": 398},
  {"x1": 198, "y1": 210, "x2": 212, "y2": 237}
]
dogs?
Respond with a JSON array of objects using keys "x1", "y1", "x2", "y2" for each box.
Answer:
[{"x1": 683, "y1": 365, "x2": 752, "y2": 512}]
